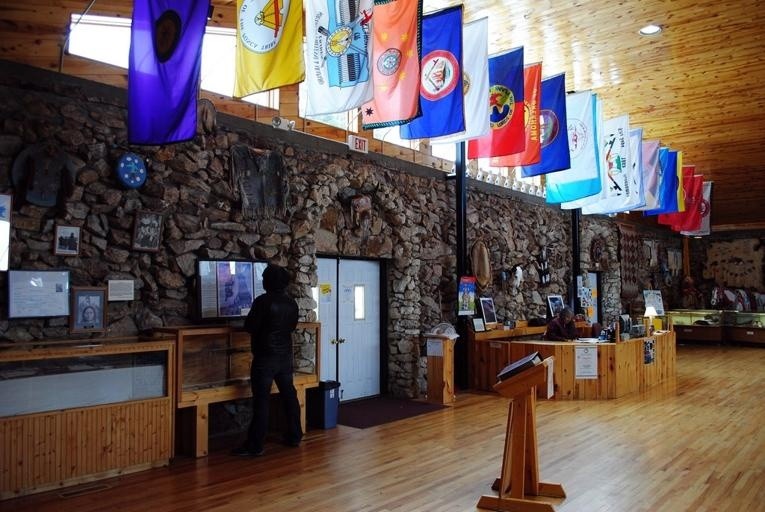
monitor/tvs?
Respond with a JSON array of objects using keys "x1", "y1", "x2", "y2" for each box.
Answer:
[
  {"x1": 496, "y1": 351, "x2": 543, "y2": 382},
  {"x1": 610, "y1": 313, "x2": 630, "y2": 343},
  {"x1": 193, "y1": 256, "x2": 272, "y2": 326},
  {"x1": 545, "y1": 294, "x2": 565, "y2": 319}
]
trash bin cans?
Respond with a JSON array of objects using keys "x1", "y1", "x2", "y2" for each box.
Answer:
[{"x1": 306, "y1": 380, "x2": 341, "y2": 430}]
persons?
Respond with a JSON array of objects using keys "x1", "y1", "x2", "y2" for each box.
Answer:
[
  {"x1": 545, "y1": 306, "x2": 578, "y2": 341},
  {"x1": 230, "y1": 264, "x2": 303, "y2": 456},
  {"x1": 80, "y1": 304, "x2": 97, "y2": 322}
]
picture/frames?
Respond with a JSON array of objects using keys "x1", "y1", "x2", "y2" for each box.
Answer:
[
  {"x1": 547, "y1": 295, "x2": 565, "y2": 318},
  {"x1": 132, "y1": 210, "x2": 163, "y2": 253},
  {"x1": 9, "y1": 267, "x2": 73, "y2": 321},
  {"x1": 54, "y1": 224, "x2": 82, "y2": 257},
  {"x1": 69, "y1": 286, "x2": 108, "y2": 333},
  {"x1": 216, "y1": 258, "x2": 255, "y2": 318}
]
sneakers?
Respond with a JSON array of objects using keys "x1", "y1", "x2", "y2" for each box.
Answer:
[
  {"x1": 288, "y1": 437, "x2": 301, "y2": 447},
  {"x1": 231, "y1": 445, "x2": 266, "y2": 457}
]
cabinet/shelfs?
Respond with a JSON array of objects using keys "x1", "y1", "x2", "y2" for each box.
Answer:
[
  {"x1": 1, "y1": 337, "x2": 176, "y2": 501},
  {"x1": 664, "y1": 309, "x2": 765, "y2": 346},
  {"x1": 142, "y1": 321, "x2": 321, "y2": 458}
]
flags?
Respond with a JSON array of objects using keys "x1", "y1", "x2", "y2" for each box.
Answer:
[
  {"x1": 399, "y1": 2, "x2": 467, "y2": 142},
  {"x1": 631, "y1": 141, "x2": 712, "y2": 235},
  {"x1": 521, "y1": 71, "x2": 609, "y2": 211},
  {"x1": 129, "y1": 1, "x2": 211, "y2": 146},
  {"x1": 233, "y1": 2, "x2": 307, "y2": 99},
  {"x1": 462, "y1": 17, "x2": 541, "y2": 169},
  {"x1": 299, "y1": 2, "x2": 374, "y2": 124},
  {"x1": 361, "y1": 0, "x2": 425, "y2": 131},
  {"x1": 582, "y1": 114, "x2": 661, "y2": 216}
]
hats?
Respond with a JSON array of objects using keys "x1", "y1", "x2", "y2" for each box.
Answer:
[{"x1": 260, "y1": 263, "x2": 291, "y2": 287}]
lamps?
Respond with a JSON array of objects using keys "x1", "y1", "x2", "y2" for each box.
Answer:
[{"x1": 643, "y1": 307, "x2": 659, "y2": 326}]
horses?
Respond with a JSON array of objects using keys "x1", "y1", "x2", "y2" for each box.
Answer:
[{"x1": 711, "y1": 283, "x2": 757, "y2": 313}]
